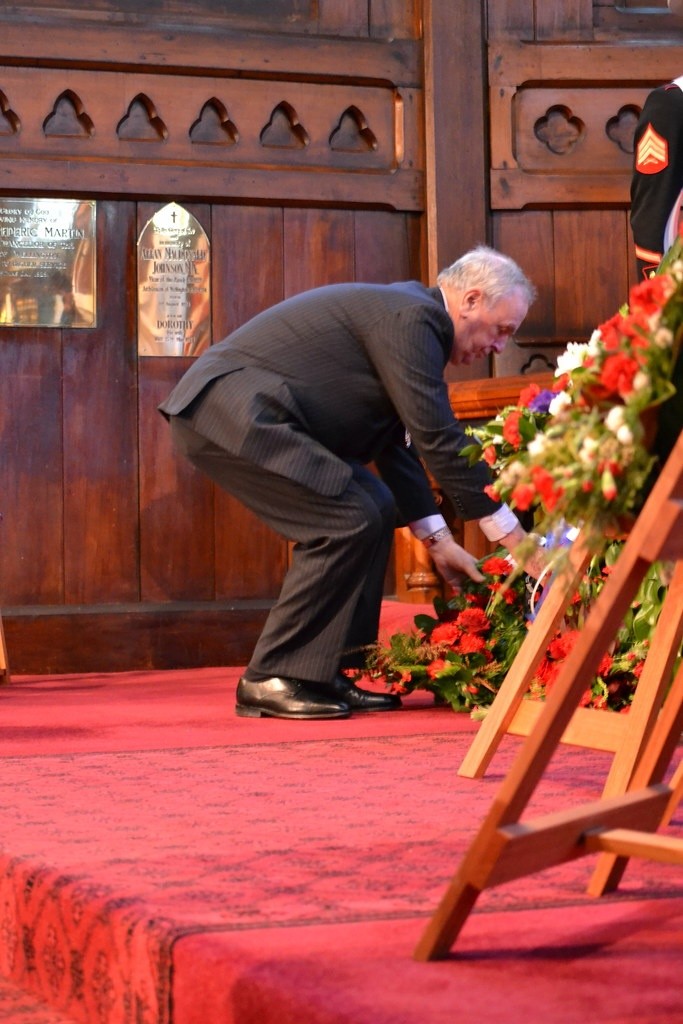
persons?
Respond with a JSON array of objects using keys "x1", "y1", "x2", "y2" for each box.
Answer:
[{"x1": 158, "y1": 246, "x2": 557, "y2": 719}]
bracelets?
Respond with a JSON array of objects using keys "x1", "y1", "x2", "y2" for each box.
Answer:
[{"x1": 423, "y1": 524, "x2": 451, "y2": 551}]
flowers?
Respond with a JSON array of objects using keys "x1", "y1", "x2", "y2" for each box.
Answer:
[{"x1": 348, "y1": 233, "x2": 682, "y2": 715}]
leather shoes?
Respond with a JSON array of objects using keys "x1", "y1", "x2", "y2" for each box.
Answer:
[{"x1": 235, "y1": 670, "x2": 402, "y2": 718}]
